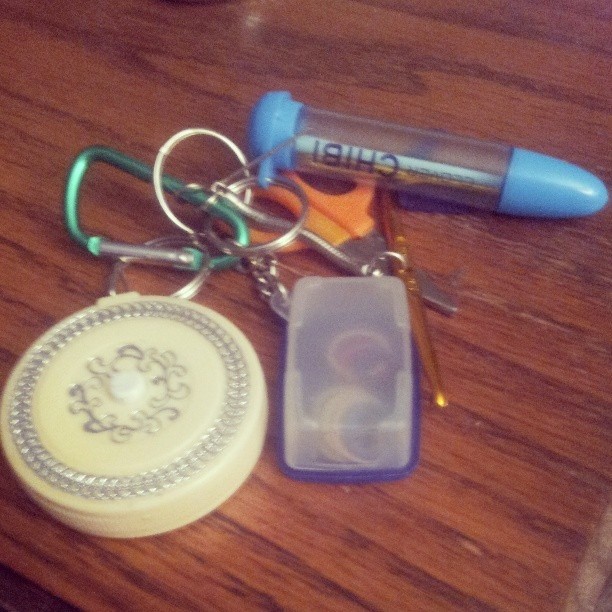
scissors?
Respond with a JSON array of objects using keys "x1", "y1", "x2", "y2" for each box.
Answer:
[{"x1": 214, "y1": 169, "x2": 459, "y2": 315}]
